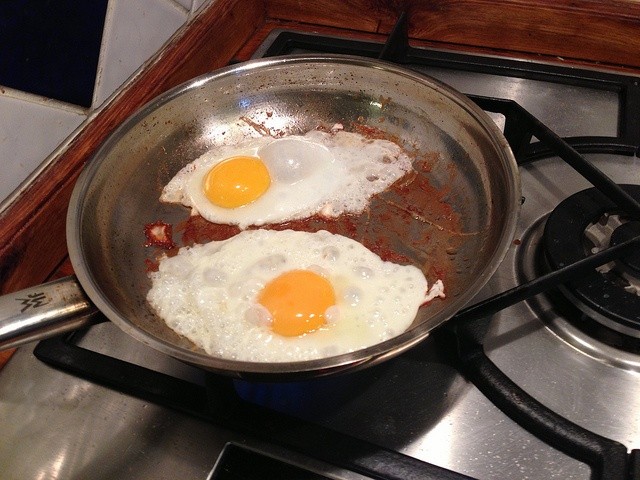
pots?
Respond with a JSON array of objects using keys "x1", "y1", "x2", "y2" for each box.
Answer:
[{"x1": 0, "y1": 54, "x2": 522, "y2": 375}]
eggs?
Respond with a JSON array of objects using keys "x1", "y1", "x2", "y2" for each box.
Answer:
[
  {"x1": 159, "y1": 131, "x2": 414, "y2": 234},
  {"x1": 146, "y1": 230, "x2": 427, "y2": 366}
]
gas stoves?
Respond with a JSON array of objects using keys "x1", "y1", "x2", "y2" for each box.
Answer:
[{"x1": 32, "y1": 26, "x2": 640, "y2": 480}]
